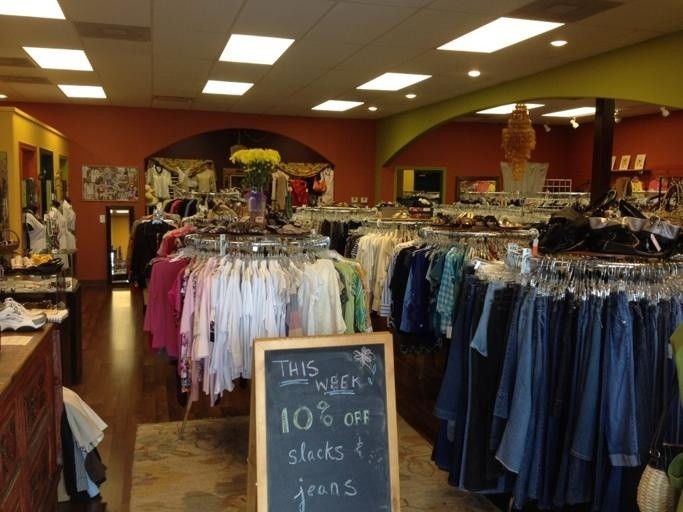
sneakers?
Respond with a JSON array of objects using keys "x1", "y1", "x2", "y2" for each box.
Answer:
[{"x1": 0, "y1": 298, "x2": 48, "y2": 333}]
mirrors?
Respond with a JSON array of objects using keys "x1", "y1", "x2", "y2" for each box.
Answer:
[{"x1": 105, "y1": 205, "x2": 135, "y2": 285}]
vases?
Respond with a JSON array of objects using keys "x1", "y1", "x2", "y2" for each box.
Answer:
[{"x1": 241, "y1": 190, "x2": 266, "y2": 223}]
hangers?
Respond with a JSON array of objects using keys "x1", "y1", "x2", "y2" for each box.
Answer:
[
  {"x1": 158, "y1": 204, "x2": 541, "y2": 261},
  {"x1": 523, "y1": 252, "x2": 682, "y2": 303}
]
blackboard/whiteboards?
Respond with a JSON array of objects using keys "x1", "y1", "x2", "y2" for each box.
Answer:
[{"x1": 255, "y1": 333, "x2": 403, "y2": 510}]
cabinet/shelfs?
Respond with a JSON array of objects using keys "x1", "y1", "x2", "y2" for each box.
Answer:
[{"x1": 0, "y1": 310, "x2": 65, "y2": 511}]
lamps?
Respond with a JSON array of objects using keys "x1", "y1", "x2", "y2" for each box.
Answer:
[
  {"x1": 659, "y1": 106, "x2": 669, "y2": 118},
  {"x1": 613, "y1": 108, "x2": 621, "y2": 123},
  {"x1": 499, "y1": 102, "x2": 535, "y2": 182},
  {"x1": 543, "y1": 122, "x2": 550, "y2": 132},
  {"x1": 568, "y1": 117, "x2": 578, "y2": 129}
]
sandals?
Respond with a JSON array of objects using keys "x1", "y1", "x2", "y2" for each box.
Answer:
[
  {"x1": 497, "y1": 214, "x2": 523, "y2": 230},
  {"x1": 430, "y1": 212, "x2": 453, "y2": 226},
  {"x1": 449, "y1": 211, "x2": 475, "y2": 228},
  {"x1": 473, "y1": 214, "x2": 501, "y2": 231}
]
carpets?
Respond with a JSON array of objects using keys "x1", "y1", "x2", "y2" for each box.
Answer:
[{"x1": 125, "y1": 413, "x2": 497, "y2": 512}]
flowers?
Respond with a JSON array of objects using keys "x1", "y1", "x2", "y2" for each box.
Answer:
[{"x1": 228, "y1": 147, "x2": 281, "y2": 192}]
